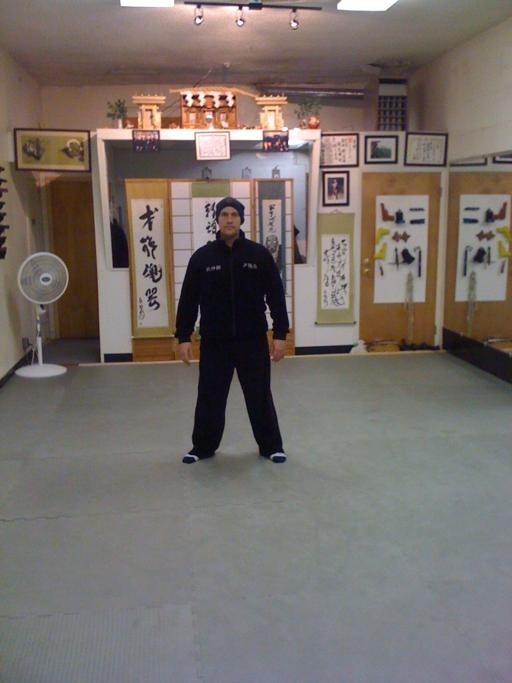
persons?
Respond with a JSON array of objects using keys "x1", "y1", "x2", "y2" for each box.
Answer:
[{"x1": 175, "y1": 197, "x2": 290, "y2": 464}]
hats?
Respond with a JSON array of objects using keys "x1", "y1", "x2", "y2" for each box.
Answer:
[{"x1": 215, "y1": 196, "x2": 245, "y2": 225}]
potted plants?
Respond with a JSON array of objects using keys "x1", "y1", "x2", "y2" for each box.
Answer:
[
  {"x1": 295, "y1": 99, "x2": 321, "y2": 128},
  {"x1": 104, "y1": 97, "x2": 127, "y2": 128}
]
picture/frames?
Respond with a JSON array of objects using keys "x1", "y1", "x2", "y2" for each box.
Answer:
[
  {"x1": 321, "y1": 131, "x2": 448, "y2": 167},
  {"x1": 14, "y1": 127, "x2": 91, "y2": 173},
  {"x1": 132, "y1": 130, "x2": 161, "y2": 154},
  {"x1": 322, "y1": 171, "x2": 350, "y2": 207},
  {"x1": 194, "y1": 131, "x2": 231, "y2": 161},
  {"x1": 451, "y1": 153, "x2": 512, "y2": 165}
]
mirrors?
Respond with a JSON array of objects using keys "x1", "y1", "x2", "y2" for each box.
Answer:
[{"x1": 443, "y1": 149, "x2": 511, "y2": 356}]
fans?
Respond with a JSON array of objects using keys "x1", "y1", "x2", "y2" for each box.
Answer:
[{"x1": 14, "y1": 252, "x2": 69, "y2": 378}]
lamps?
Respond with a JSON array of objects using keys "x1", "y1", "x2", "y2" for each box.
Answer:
[{"x1": 185, "y1": 1, "x2": 322, "y2": 30}]
